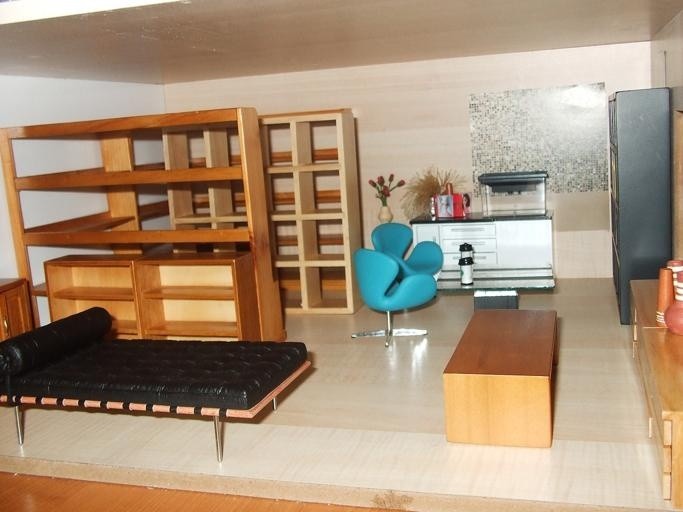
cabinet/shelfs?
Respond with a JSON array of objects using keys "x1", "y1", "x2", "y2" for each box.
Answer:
[
  {"x1": 608, "y1": 87, "x2": 672, "y2": 325},
  {"x1": 629, "y1": 279, "x2": 683, "y2": 509},
  {"x1": 410, "y1": 210, "x2": 555, "y2": 290},
  {"x1": 161, "y1": 107, "x2": 362, "y2": 316},
  {"x1": 1, "y1": 108, "x2": 286, "y2": 341},
  {"x1": 44, "y1": 254, "x2": 262, "y2": 342},
  {"x1": 1, "y1": 277, "x2": 36, "y2": 340}
]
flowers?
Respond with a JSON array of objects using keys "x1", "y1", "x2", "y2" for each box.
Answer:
[{"x1": 369, "y1": 174, "x2": 405, "y2": 206}]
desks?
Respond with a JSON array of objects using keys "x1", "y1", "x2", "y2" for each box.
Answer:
[{"x1": 443, "y1": 309, "x2": 558, "y2": 448}]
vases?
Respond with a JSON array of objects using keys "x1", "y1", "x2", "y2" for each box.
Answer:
[{"x1": 378, "y1": 206, "x2": 393, "y2": 223}]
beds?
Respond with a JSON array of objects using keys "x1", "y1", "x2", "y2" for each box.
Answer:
[{"x1": 0, "y1": 340, "x2": 311, "y2": 461}]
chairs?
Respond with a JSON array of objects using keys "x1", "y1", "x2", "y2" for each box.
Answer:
[
  {"x1": 350, "y1": 247, "x2": 437, "y2": 347},
  {"x1": 371, "y1": 223, "x2": 443, "y2": 284}
]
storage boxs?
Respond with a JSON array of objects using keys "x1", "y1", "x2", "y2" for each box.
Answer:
[{"x1": 478, "y1": 171, "x2": 548, "y2": 218}]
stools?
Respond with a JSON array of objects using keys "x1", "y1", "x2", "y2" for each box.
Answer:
[{"x1": 474, "y1": 290, "x2": 518, "y2": 309}]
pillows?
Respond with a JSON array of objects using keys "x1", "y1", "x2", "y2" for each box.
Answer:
[{"x1": 0, "y1": 307, "x2": 112, "y2": 377}]
